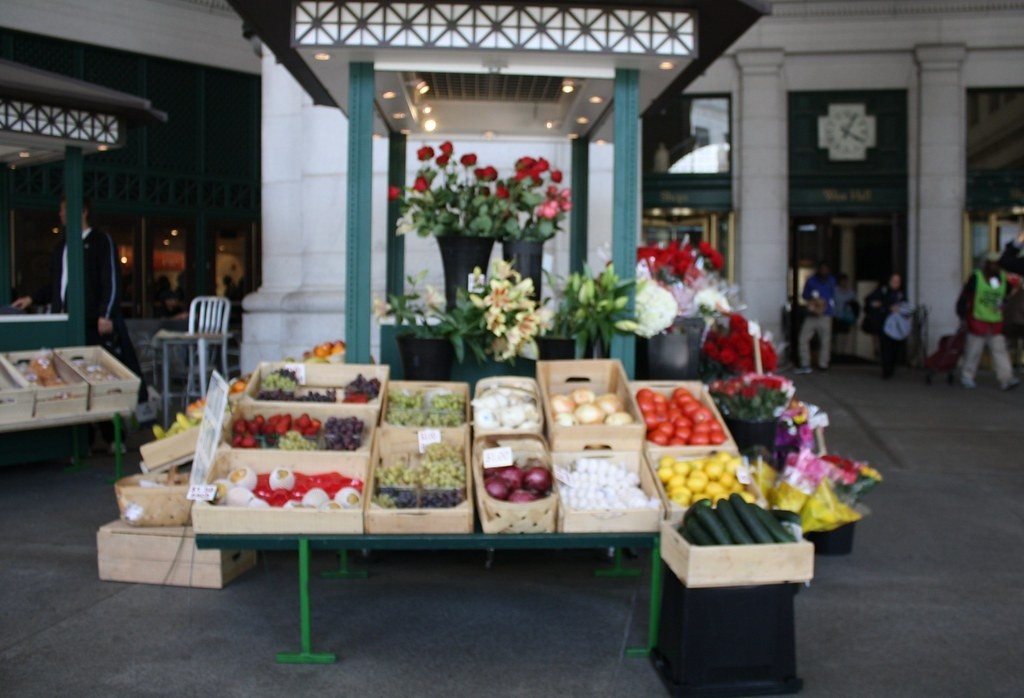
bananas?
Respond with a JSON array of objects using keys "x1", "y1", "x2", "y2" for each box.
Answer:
[{"x1": 153, "y1": 411, "x2": 200, "y2": 438}]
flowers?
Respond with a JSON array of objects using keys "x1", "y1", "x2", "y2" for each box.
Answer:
[
  {"x1": 491, "y1": 157, "x2": 572, "y2": 243},
  {"x1": 635, "y1": 240, "x2": 881, "y2": 529},
  {"x1": 373, "y1": 261, "x2": 553, "y2": 363},
  {"x1": 543, "y1": 264, "x2": 646, "y2": 358},
  {"x1": 388, "y1": 141, "x2": 511, "y2": 237}
]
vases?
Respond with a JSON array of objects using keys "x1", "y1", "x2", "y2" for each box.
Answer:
[
  {"x1": 532, "y1": 335, "x2": 577, "y2": 363},
  {"x1": 501, "y1": 239, "x2": 542, "y2": 303},
  {"x1": 435, "y1": 233, "x2": 496, "y2": 313},
  {"x1": 398, "y1": 333, "x2": 452, "y2": 380}
]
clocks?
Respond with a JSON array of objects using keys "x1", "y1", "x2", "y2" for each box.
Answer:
[{"x1": 818, "y1": 103, "x2": 878, "y2": 161}]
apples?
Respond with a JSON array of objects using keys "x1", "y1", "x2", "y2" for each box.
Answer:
[{"x1": 208, "y1": 467, "x2": 361, "y2": 511}]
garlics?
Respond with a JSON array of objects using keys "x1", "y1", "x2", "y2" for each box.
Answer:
[{"x1": 557, "y1": 455, "x2": 657, "y2": 509}]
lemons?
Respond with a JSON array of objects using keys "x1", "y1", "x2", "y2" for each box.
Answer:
[{"x1": 657, "y1": 450, "x2": 756, "y2": 505}]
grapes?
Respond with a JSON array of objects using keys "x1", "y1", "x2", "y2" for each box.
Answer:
[
  {"x1": 257, "y1": 368, "x2": 381, "y2": 403},
  {"x1": 277, "y1": 415, "x2": 364, "y2": 452},
  {"x1": 371, "y1": 390, "x2": 469, "y2": 508}
]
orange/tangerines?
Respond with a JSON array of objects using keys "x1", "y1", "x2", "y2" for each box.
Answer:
[
  {"x1": 186, "y1": 381, "x2": 248, "y2": 412},
  {"x1": 303, "y1": 340, "x2": 343, "y2": 359}
]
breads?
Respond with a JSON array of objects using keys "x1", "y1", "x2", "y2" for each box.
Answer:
[{"x1": 24, "y1": 357, "x2": 74, "y2": 400}]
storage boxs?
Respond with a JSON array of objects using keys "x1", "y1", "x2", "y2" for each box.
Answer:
[
  {"x1": 0, "y1": 367, "x2": 34, "y2": 419},
  {"x1": 3, "y1": 349, "x2": 89, "y2": 417},
  {"x1": 659, "y1": 522, "x2": 815, "y2": 587},
  {"x1": 95, "y1": 352, "x2": 771, "y2": 589},
  {"x1": 53, "y1": 344, "x2": 142, "y2": 415}
]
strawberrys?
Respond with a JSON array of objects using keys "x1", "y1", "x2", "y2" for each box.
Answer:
[{"x1": 232, "y1": 412, "x2": 321, "y2": 449}]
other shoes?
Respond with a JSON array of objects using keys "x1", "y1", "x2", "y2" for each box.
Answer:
[
  {"x1": 109, "y1": 443, "x2": 126, "y2": 456},
  {"x1": 1001, "y1": 379, "x2": 1020, "y2": 391},
  {"x1": 820, "y1": 366, "x2": 830, "y2": 375},
  {"x1": 793, "y1": 366, "x2": 811, "y2": 374},
  {"x1": 962, "y1": 380, "x2": 976, "y2": 389}
]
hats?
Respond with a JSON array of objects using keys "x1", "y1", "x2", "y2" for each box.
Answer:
[{"x1": 984, "y1": 250, "x2": 1001, "y2": 261}]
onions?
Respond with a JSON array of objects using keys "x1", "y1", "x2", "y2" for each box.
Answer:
[
  {"x1": 551, "y1": 389, "x2": 634, "y2": 449},
  {"x1": 485, "y1": 462, "x2": 553, "y2": 503}
]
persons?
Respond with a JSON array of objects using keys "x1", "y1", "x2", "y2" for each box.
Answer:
[
  {"x1": 124, "y1": 272, "x2": 240, "y2": 322},
  {"x1": 12, "y1": 195, "x2": 148, "y2": 454},
  {"x1": 865, "y1": 273, "x2": 904, "y2": 379},
  {"x1": 954, "y1": 230, "x2": 1024, "y2": 391},
  {"x1": 793, "y1": 261, "x2": 860, "y2": 374}
]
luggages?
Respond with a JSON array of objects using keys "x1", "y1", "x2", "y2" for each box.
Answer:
[{"x1": 925, "y1": 326, "x2": 967, "y2": 386}]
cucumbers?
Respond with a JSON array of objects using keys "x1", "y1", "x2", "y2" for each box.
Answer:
[{"x1": 680, "y1": 493, "x2": 802, "y2": 546}]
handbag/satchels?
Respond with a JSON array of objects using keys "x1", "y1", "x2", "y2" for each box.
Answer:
[{"x1": 863, "y1": 310, "x2": 876, "y2": 334}]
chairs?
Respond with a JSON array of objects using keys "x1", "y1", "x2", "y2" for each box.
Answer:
[{"x1": 163, "y1": 295, "x2": 231, "y2": 428}]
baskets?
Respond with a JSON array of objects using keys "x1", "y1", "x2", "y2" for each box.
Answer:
[
  {"x1": 115, "y1": 474, "x2": 192, "y2": 527},
  {"x1": 472, "y1": 376, "x2": 559, "y2": 532}
]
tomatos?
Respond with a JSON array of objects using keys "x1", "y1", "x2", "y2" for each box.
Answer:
[{"x1": 636, "y1": 388, "x2": 726, "y2": 446}]
